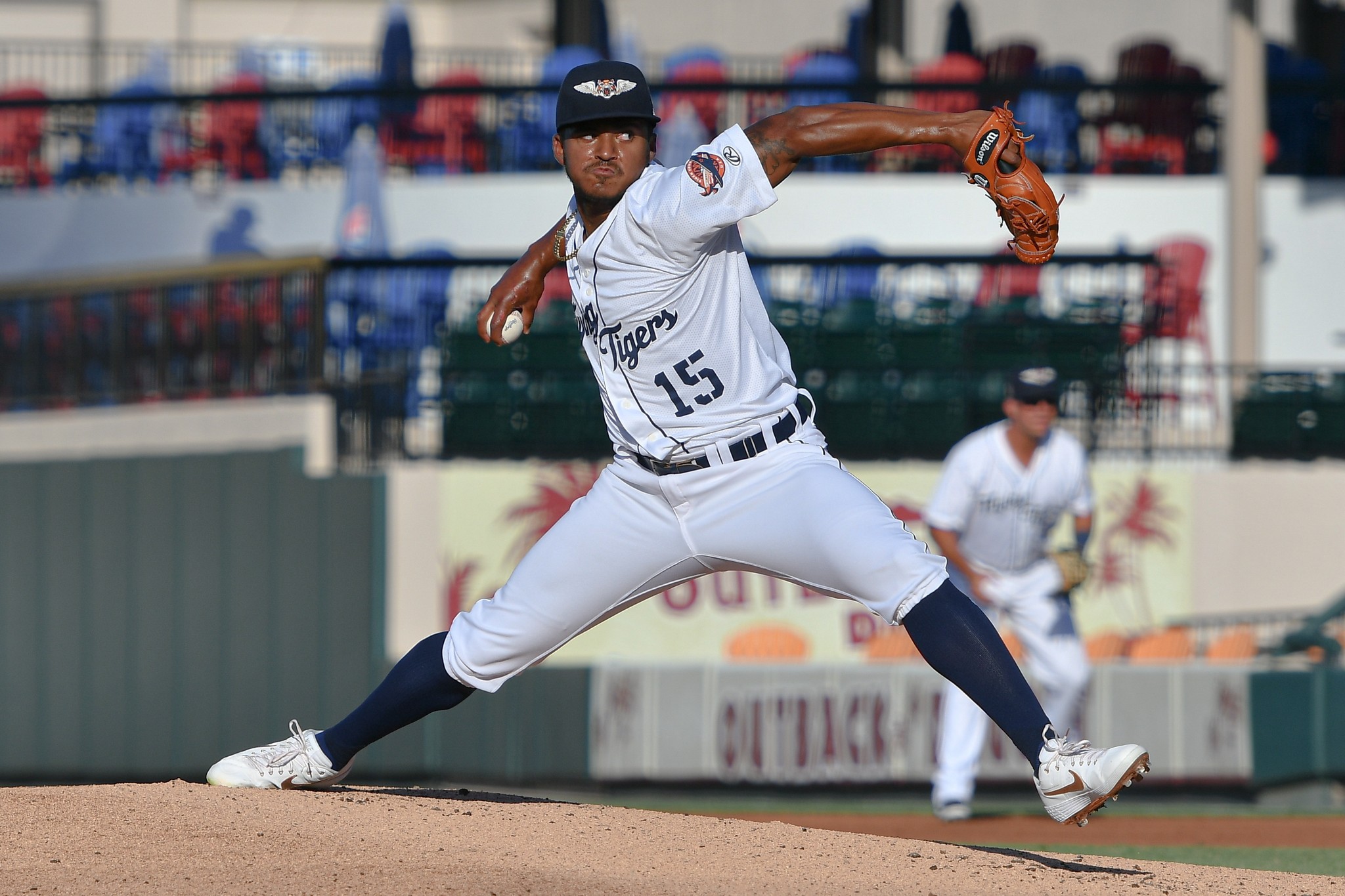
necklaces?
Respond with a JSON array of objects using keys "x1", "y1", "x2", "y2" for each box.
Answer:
[{"x1": 554, "y1": 208, "x2": 583, "y2": 262}]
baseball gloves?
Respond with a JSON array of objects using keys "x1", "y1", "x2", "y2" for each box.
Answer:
[{"x1": 964, "y1": 108, "x2": 1060, "y2": 263}]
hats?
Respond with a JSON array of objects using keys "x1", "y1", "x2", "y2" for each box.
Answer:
[
  {"x1": 556, "y1": 59, "x2": 661, "y2": 132},
  {"x1": 1013, "y1": 364, "x2": 1062, "y2": 402}
]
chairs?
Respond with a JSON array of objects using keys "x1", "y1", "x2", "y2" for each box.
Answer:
[
  {"x1": 0, "y1": 37, "x2": 1210, "y2": 188},
  {"x1": 330, "y1": 237, "x2": 1217, "y2": 420},
  {"x1": 721, "y1": 624, "x2": 1259, "y2": 665}
]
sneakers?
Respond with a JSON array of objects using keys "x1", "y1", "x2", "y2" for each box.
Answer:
[
  {"x1": 1032, "y1": 725, "x2": 1153, "y2": 827},
  {"x1": 206, "y1": 721, "x2": 356, "y2": 789}
]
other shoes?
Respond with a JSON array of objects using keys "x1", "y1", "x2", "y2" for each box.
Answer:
[{"x1": 932, "y1": 798, "x2": 972, "y2": 820}]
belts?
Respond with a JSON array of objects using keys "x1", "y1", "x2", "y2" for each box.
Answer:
[{"x1": 635, "y1": 400, "x2": 808, "y2": 477}]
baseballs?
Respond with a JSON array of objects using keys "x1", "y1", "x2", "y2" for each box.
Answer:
[{"x1": 486, "y1": 309, "x2": 523, "y2": 344}]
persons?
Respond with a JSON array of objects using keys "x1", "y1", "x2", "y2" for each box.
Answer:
[
  {"x1": 205, "y1": 59, "x2": 1151, "y2": 827},
  {"x1": 923, "y1": 362, "x2": 1091, "y2": 822}
]
